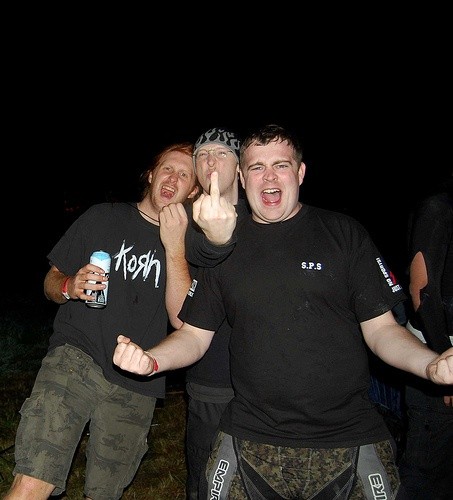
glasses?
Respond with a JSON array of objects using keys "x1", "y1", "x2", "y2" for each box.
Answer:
[{"x1": 196, "y1": 147, "x2": 233, "y2": 159}]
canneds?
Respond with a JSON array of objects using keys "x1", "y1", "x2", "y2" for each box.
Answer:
[{"x1": 85, "y1": 249, "x2": 111, "y2": 308}]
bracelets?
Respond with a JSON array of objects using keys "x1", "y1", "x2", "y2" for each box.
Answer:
[
  {"x1": 145, "y1": 351, "x2": 158, "y2": 377},
  {"x1": 60, "y1": 276, "x2": 73, "y2": 300}
]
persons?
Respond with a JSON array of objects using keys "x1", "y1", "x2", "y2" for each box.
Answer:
[
  {"x1": 0, "y1": 143, "x2": 203, "y2": 500},
  {"x1": 184, "y1": 128, "x2": 250, "y2": 500},
  {"x1": 403, "y1": 196, "x2": 453, "y2": 500},
  {"x1": 112, "y1": 125, "x2": 453, "y2": 500}
]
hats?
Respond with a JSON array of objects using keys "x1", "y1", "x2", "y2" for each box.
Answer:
[{"x1": 193, "y1": 128, "x2": 240, "y2": 173}]
